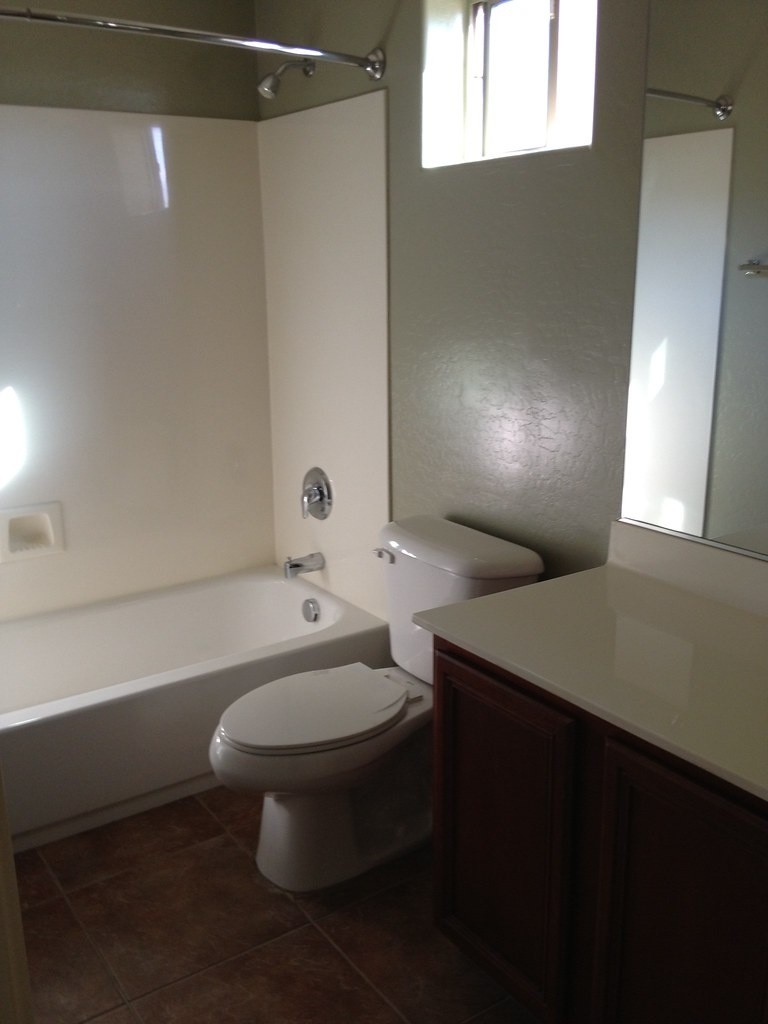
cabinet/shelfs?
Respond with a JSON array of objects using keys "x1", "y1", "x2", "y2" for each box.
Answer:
[{"x1": 431, "y1": 634, "x2": 768, "y2": 1023}]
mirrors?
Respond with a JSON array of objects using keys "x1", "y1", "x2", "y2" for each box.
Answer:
[{"x1": 616, "y1": 0, "x2": 768, "y2": 558}]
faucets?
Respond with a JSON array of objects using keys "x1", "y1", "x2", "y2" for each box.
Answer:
[{"x1": 283, "y1": 550, "x2": 324, "y2": 581}]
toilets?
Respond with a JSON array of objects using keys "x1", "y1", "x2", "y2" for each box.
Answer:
[{"x1": 209, "y1": 513, "x2": 542, "y2": 885}]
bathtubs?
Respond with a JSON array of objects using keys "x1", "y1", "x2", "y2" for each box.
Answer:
[{"x1": 0, "y1": 563, "x2": 390, "y2": 832}]
